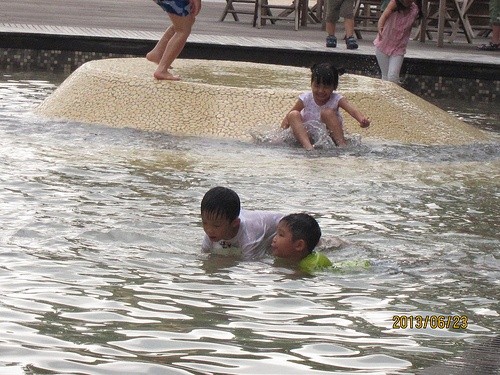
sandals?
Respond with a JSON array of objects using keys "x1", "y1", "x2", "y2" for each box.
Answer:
[{"x1": 478, "y1": 41, "x2": 500, "y2": 51}]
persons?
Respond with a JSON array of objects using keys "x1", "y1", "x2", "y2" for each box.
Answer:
[
  {"x1": 271, "y1": 212, "x2": 376, "y2": 276},
  {"x1": 478, "y1": 0, "x2": 499, "y2": 49},
  {"x1": 146, "y1": 0, "x2": 201, "y2": 80},
  {"x1": 374, "y1": 1, "x2": 418, "y2": 85},
  {"x1": 200, "y1": 185, "x2": 287, "y2": 259},
  {"x1": 327, "y1": 0, "x2": 358, "y2": 49},
  {"x1": 279, "y1": 61, "x2": 369, "y2": 152}
]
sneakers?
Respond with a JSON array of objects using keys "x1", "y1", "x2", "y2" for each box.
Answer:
[
  {"x1": 325, "y1": 36, "x2": 337, "y2": 47},
  {"x1": 345, "y1": 35, "x2": 358, "y2": 49}
]
